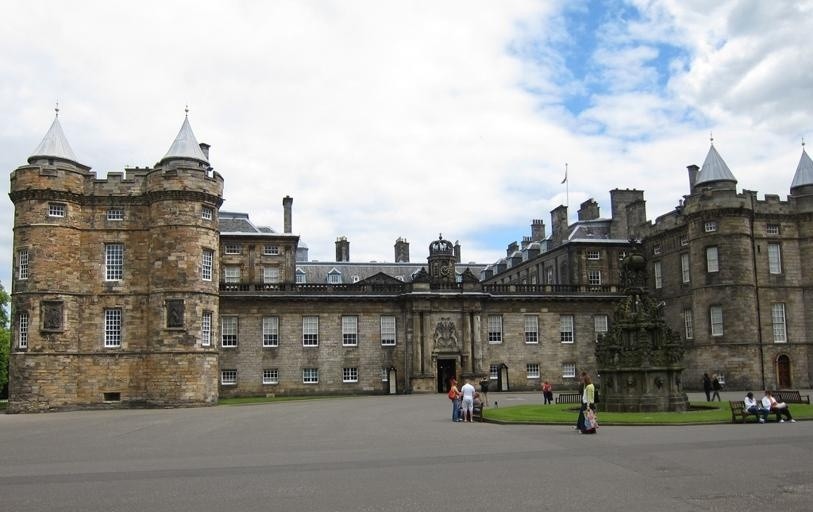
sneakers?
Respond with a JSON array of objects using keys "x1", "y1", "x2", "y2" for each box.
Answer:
[
  {"x1": 575, "y1": 426, "x2": 592, "y2": 433},
  {"x1": 757, "y1": 417, "x2": 796, "y2": 424},
  {"x1": 453, "y1": 418, "x2": 474, "y2": 424}
]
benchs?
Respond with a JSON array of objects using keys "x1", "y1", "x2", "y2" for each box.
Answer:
[{"x1": 729, "y1": 390, "x2": 810, "y2": 424}]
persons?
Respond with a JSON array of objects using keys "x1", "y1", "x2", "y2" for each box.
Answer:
[
  {"x1": 542, "y1": 380, "x2": 552, "y2": 405},
  {"x1": 743, "y1": 391, "x2": 769, "y2": 425},
  {"x1": 441, "y1": 374, "x2": 490, "y2": 423},
  {"x1": 710, "y1": 375, "x2": 721, "y2": 402},
  {"x1": 761, "y1": 390, "x2": 796, "y2": 423},
  {"x1": 578, "y1": 375, "x2": 597, "y2": 433},
  {"x1": 574, "y1": 372, "x2": 587, "y2": 431},
  {"x1": 701, "y1": 373, "x2": 711, "y2": 401}
]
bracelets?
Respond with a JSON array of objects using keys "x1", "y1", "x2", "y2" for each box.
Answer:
[{"x1": 586, "y1": 406, "x2": 589, "y2": 407}]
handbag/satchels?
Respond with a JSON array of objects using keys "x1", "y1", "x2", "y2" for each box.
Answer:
[
  {"x1": 583, "y1": 409, "x2": 600, "y2": 431},
  {"x1": 448, "y1": 386, "x2": 456, "y2": 400}
]
